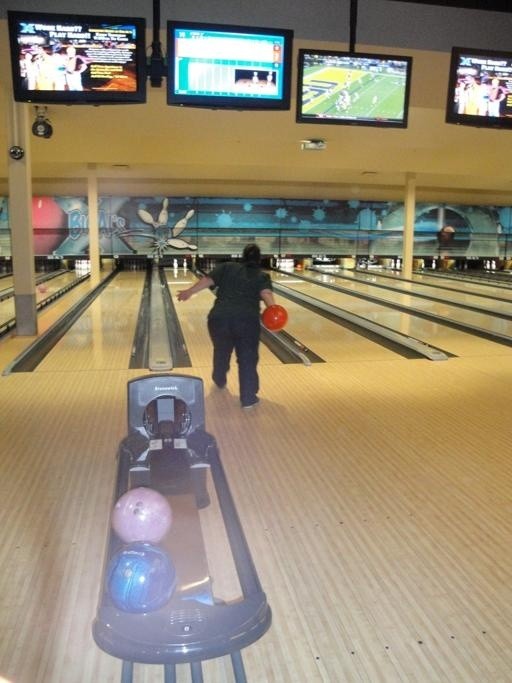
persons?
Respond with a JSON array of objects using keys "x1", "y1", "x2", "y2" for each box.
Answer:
[
  {"x1": 23, "y1": 42, "x2": 89, "y2": 91},
  {"x1": 454, "y1": 71, "x2": 508, "y2": 116},
  {"x1": 301, "y1": 52, "x2": 407, "y2": 121},
  {"x1": 175, "y1": 243, "x2": 277, "y2": 410}
]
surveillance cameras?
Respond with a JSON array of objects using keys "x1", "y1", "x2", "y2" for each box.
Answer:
[{"x1": 9, "y1": 145, "x2": 23, "y2": 160}]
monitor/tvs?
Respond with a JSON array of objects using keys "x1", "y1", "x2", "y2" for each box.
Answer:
[
  {"x1": 296, "y1": 48, "x2": 413, "y2": 129},
  {"x1": 6, "y1": 9, "x2": 147, "y2": 104},
  {"x1": 445, "y1": 46, "x2": 512, "y2": 130},
  {"x1": 167, "y1": 19, "x2": 294, "y2": 111}
]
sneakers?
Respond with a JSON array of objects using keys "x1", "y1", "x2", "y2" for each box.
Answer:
[{"x1": 243, "y1": 396, "x2": 265, "y2": 409}]
